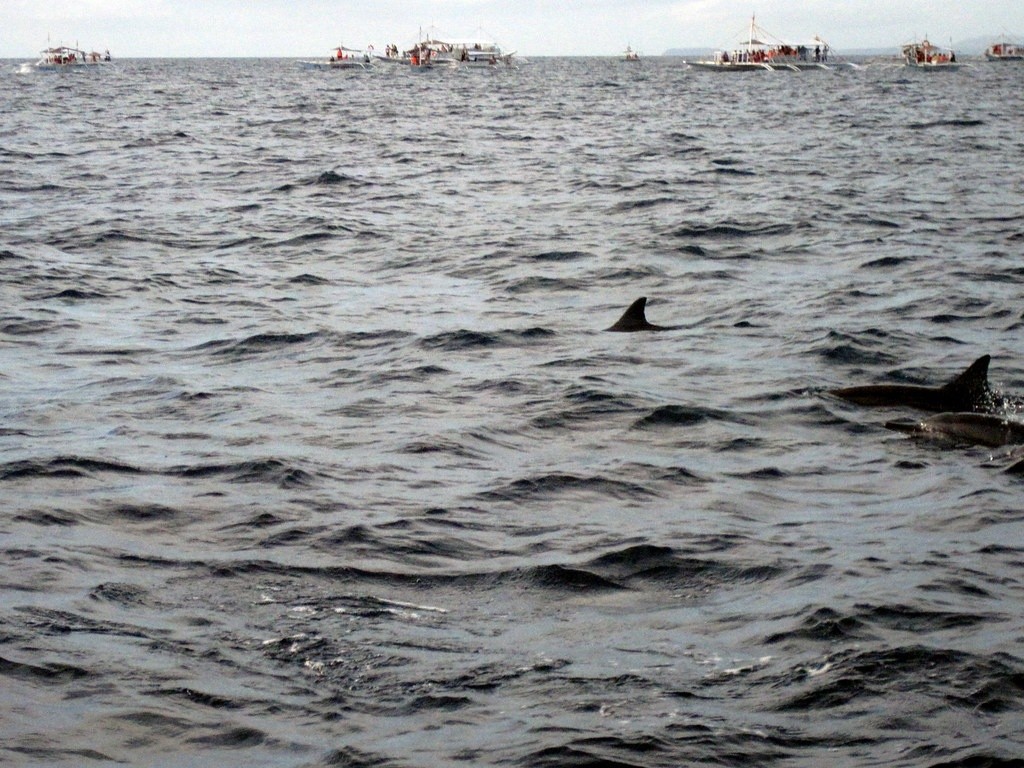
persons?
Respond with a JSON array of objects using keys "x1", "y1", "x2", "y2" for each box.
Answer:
[
  {"x1": 365, "y1": 58, "x2": 370, "y2": 63},
  {"x1": 798, "y1": 45, "x2": 807, "y2": 54},
  {"x1": 942, "y1": 54, "x2": 948, "y2": 62},
  {"x1": 337, "y1": 48, "x2": 342, "y2": 60},
  {"x1": 754, "y1": 50, "x2": 765, "y2": 62},
  {"x1": 781, "y1": 46, "x2": 791, "y2": 55},
  {"x1": 105, "y1": 51, "x2": 110, "y2": 62},
  {"x1": 722, "y1": 53, "x2": 729, "y2": 62},
  {"x1": 822, "y1": 46, "x2": 828, "y2": 62},
  {"x1": 390, "y1": 44, "x2": 398, "y2": 58},
  {"x1": 385, "y1": 45, "x2": 389, "y2": 57},
  {"x1": 815, "y1": 46, "x2": 820, "y2": 61},
  {"x1": 461, "y1": 50, "x2": 465, "y2": 61},
  {"x1": 930, "y1": 53, "x2": 938, "y2": 63},
  {"x1": 54, "y1": 54, "x2": 77, "y2": 64},
  {"x1": 950, "y1": 55, "x2": 955, "y2": 62},
  {"x1": 937, "y1": 53, "x2": 943, "y2": 62},
  {"x1": 492, "y1": 55, "x2": 496, "y2": 62},
  {"x1": 330, "y1": 56, "x2": 334, "y2": 62}
]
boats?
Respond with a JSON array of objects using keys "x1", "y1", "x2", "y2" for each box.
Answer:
[
  {"x1": 900, "y1": 32, "x2": 960, "y2": 72},
  {"x1": 31, "y1": 34, "x2": 115, "y2": 74},
  {"x1": 617, "y1": 45, "x2": 640, "y2": 62},
  {"x1": 681, "y1": 11, "x2": 862, "y2": 74},
  {"x1": 983, "y1": 34, "x2": 1024, "y2": 61},
  {"x1": 297, "y1": 25, "x2": 518, "y2": 68}
]
